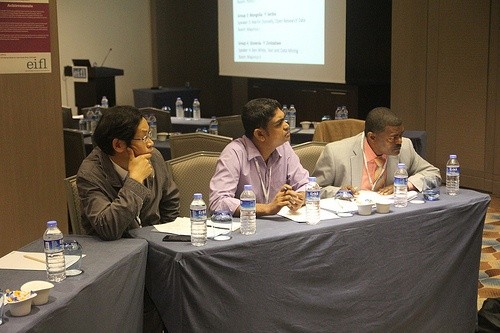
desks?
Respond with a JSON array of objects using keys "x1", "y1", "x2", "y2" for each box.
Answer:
[
  {"x1": 0, "y1": 233, "x2": 149, "y2": 333},
  {"x1": 121, "y1": 186, "x2": 491, "y2": 333}
]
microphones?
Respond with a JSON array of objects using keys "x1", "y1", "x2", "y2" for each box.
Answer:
[{"x1": 101, "y1": 48, "x2": 112, "y2": 66}]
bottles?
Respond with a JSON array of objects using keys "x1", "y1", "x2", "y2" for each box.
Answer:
[
  {"x1": 176, "y1": 98, "x2": 219, "y2": 136},
  {"x1": 86, "y1": 96, "x2": 109, "y2": 135},
  {"x1": 240, "y1": 186, "x2": 256, "y2": 235},
  {"x1": 189, "y1": 194, "x2": 208, "y2": 247},
  {"x1": 304, "y1": 177, "x2": 321, "y2": 225},
  {"x1": 43, "y1": 221, "x2": 65, "y2": 283},
  {"x1": 394, "y1": 164, "x2": 409, "y2": 207},
  {"x1": 144, "y1": 114, "x2": 157, "y2": 140},
  {"x1": 281, "y1": 103, "x2": 348, "y2": 129},
  {"x1": 447, "y1": 155, "x2": 460, "y2": 195}
]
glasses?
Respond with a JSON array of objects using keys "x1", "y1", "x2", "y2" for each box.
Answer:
[
  {"x1": 120, "y1": 130, "x2": 152, "y2": 144},
  {"x1": 377, "y1": 133, "x2": 402, "y2": 143}
]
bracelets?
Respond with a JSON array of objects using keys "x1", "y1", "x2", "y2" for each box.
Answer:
[{"x1": 407, "y1": 185, "x2": 409, "y2": 191}]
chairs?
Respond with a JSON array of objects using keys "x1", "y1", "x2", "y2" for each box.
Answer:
[{"x1": 60, "y1": 105, "x2": 366, "y2": 235}]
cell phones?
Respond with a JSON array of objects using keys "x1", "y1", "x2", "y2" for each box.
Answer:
[{"x1": 163, "y1": 235, "x2": 192, "y2": 241}]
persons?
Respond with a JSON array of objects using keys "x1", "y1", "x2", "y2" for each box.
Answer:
[
  {"x1": 311, "y1": 107, "x2": 442, "y2": 196},
  {"x1": 207, "y1": 98, "x2": 315, "y2": 218},
  {"x1": 76, "y1": 106, "x2": 181, "y2": 333}
]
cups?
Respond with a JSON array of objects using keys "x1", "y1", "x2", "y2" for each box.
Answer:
[
  {"x1": 355, "y1": 198, "x2": 393, "y2": 214},
  {"x1": 300, "y1": 121, "x2": 311, "y2": 129},
  {"x1": 312, "y1": 121, "x2": 321, "y2": 129},
  {"x1": 423, "y1": 173, "x2": 441, "y2": 201},
  {"x1": 211, "y1": 209, "x2": 233, "y2": 241},
  {"x1": 334, "y1": 189, "x2": 355, "y2": 217},
  {"x1": 156, "y1": 132, "x2": 181, "y2": 141},
  {"x1": 0, "y1": 290, "x2": 37, "y2": 325},
  {"x1": 64, "y1": 241, "x2": 84, "y2": 276},
  {"x1": 20, "y1": 280, "x2": 54, "y2": 306}
]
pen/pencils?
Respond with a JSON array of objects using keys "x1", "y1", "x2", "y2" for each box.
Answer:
[
  {"x1": 24, "y1": 254, "x2": 46, "y2": 262},
  {"x1": 207, "y1": 225, "x2": 230, "y2": 230},
  {"x1": 283, "y1": 186, "x2": 287, "y2": 190},
  {"x1": 348, "y1": 187, "x2": 358, "y2": 191}
]
